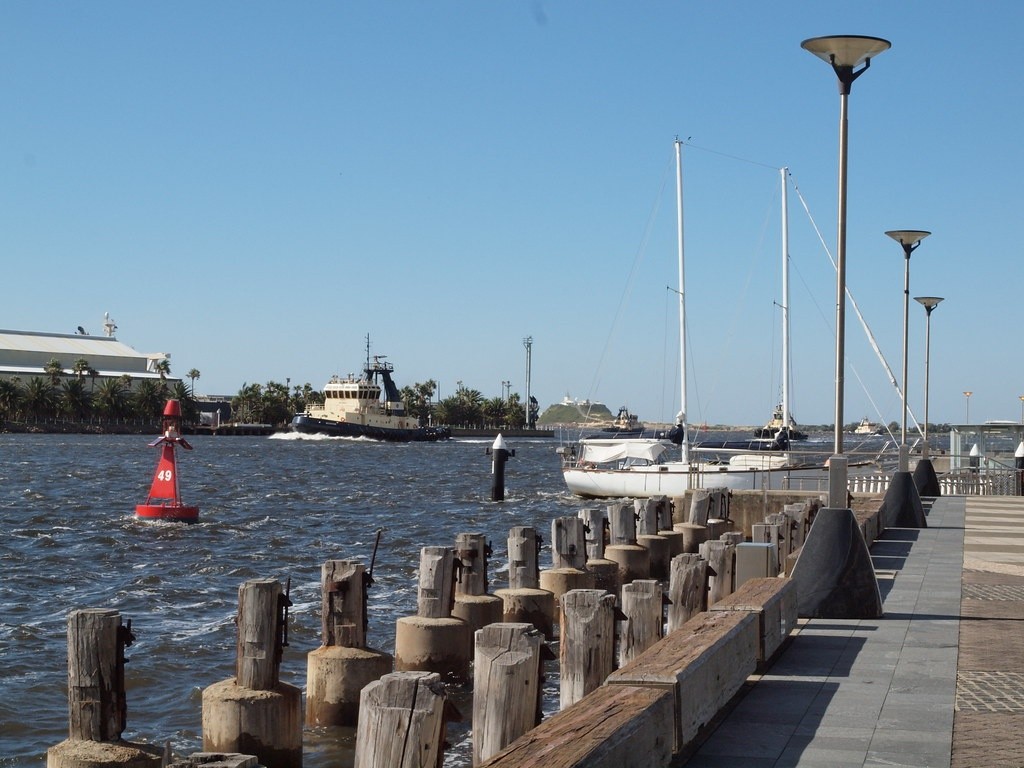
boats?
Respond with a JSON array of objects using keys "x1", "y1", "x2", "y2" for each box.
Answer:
[
  {"x1": 848, "y1": 415, "x2": 884, "y2": 436},
  {"x1": 753, "y1": 381, "x2": 809, "y2": 443},
  {"x1": 586, "y1": 406, "x2": 690, "y2": 439},
  {"x1": 287, "y1": 332, "x2": 453, "y2": 442}
]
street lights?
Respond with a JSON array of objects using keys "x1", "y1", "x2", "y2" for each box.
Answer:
[
  {"x1": 962, "y1": 391, "x2": 974, "y2": 424},
  {"x1": 913, "y1": 295, "x2": 945, "y2": 496},
  {"x1": 1018, "y1": 395, "x2": 1024, "y2": 426},
  {"x1": 791, "y1": 34, "x2": 894, "y2": 621},
  {"x1": 876, "y1": 229, "x2": 930, "y2": 530}
]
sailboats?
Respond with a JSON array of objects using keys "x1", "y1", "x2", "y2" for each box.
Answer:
[{"x1": 552, "y1": 135, "x2": 1024, "y2": 499}]
